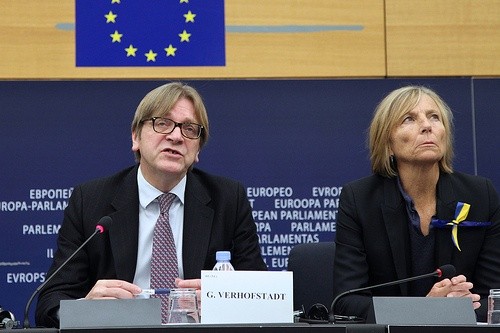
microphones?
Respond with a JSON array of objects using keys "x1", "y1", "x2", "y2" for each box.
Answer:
[
  {"x1": 327, "y1": 265, "x2": 456, "y2": 322},
  {"x1": 23, "y1": 216, "x2": 112, "y2": 329}
]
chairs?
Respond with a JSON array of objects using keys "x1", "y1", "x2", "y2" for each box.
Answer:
[{"x1": 289, "y1": 241, "x2": 335, "y2": 309}]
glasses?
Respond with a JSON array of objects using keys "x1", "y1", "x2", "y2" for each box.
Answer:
[{"x1": 142, "y1": 117, "x2": 203, "y2": 139}]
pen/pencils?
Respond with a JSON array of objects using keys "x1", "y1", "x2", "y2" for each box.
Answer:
[{"x1": 132, "y1": 288, "x2": 198, "y2": 296}]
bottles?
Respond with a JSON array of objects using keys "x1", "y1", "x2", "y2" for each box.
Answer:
[{"x1": 212, "y1": 250, "x2": 235, "y2": 271}]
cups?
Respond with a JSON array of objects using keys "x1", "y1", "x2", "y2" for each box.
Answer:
[
  {"x1": 487, "y1": 289, "x2": 500, "y2": 327},
  {"x1": 167, "y1": 288, "x2": 199, "y2": 323}
]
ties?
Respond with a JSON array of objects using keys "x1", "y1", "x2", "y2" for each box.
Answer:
[{"x1": 149, "y1": 194, "x2": 179, "y2": 324}]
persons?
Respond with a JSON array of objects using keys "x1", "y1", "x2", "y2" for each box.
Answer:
[
  {"x1": 35, "y1": 82, "x2": 269, "y2": 329},
  {"x1": 331, "y1": 87, "x2": 500, "y2": 324}
]
